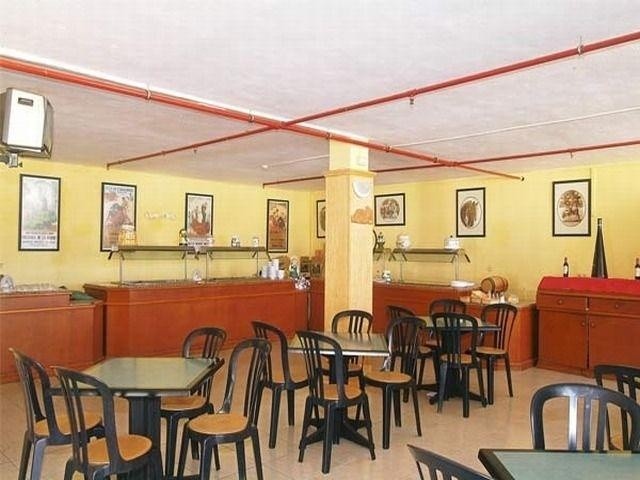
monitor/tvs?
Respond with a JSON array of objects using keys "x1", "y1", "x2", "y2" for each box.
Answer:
[{"x1": 0, "y1": 86, "x2": 55, "y2": 159}]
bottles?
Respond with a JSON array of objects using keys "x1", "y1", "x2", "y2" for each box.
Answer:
[
  {"x1": 563, "y1": 257, "x2": 569, "y2": 277},
  {"x1": 634, "y1": 257, "x2": 640, "y2": 279}
]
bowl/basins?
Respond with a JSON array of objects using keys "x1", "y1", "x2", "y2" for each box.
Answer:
[{"x1": 261, "y1": 258, "x2": 285, "y2": 281}]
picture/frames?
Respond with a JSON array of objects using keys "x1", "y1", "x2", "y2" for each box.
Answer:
[
  {"x1": 265, "y1": 198, "x2": 291, "y2": 254},
  {"x1": 16, "y1": 172, "x2": 62, "y2": 252},
  {"x1": 185, "y1": 191, "x2": 214, "y2": 246},
  {"x1": 315, "y1": 198, "x2": 326, "y2": 239},
  {"x1": 454, "y1": 186, "x2": 487, "y2": 238},
  {"x1": 550, "y1": 178, "x2": 593, "y2": 239},
  {"x1": 99, "y1": 181, "x2": 138, "y2": 252},
  {"x1": 373, "y1": 191, "x2": 407, "y2": 228}
]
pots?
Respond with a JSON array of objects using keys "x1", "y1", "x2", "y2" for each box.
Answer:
[{"x1": 443, "y1": 235, "x2": 460, "y2": 251}]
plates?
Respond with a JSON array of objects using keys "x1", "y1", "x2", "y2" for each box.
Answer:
[{"x1": 451, "y1": 281, "x2": 468, "y2": 287}]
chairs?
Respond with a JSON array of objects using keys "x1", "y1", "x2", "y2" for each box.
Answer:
[
  {"x1": 595, "y1": 365, "x2": 639, "y2": 450},
  {"x1": 159, "y1": 327, "x2": 226, "y2": 476},
  {"x1": 250, "y1": 309, "x2": 422, "y2": 473},
  {"x1": 8, "y1": 346, "x2": 105, "y2": 479},
  {"x1": 408, "y1": 443, "x2": 490, "y2": 479},
  {"x1": 50, "y1": 366, "x2": 164, "y2": 480},
  {"x1": 386, "y1": 298, "x2": 519, "y2": 420},
  {"x1": 177, "y1": 338, "x2": 272, "y2": 478},
  {"x1": 529, "y1": 382, "x2": 640, "y2": 453}
]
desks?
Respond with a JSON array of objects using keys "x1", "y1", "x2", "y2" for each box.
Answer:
[
  {"x1": 49, "y1": 358, "x2": 225, "y2": 478},
  {"x1": 83, "y1": 278, "x2": 310, "y2": 360},
  {"x1": 477, "y1": 449, "x2": 639, "y2": 479},
  {"x1": 286, "y1": 331, "x2": 390, "y2": 432},
  {"x1": 312, "y1": 279, "x2": 470, "y2": 338}
]
